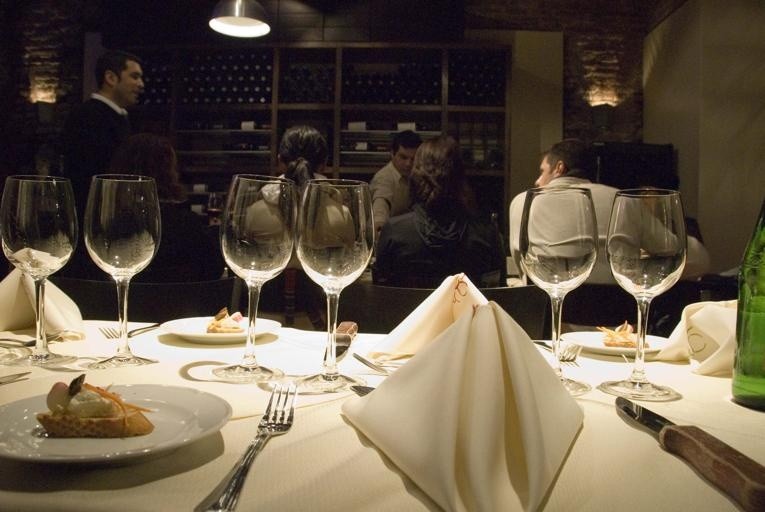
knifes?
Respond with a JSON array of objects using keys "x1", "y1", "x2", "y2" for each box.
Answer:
[
  {"x1": 0, "y1": 369, "x2": 30, "y2": 388},
  {"x1": 612, "y1": 395, "x2": 764, "y2": 511},
  {"x1": 325, "y1": 318, "x2": 360, "y2": 365}
]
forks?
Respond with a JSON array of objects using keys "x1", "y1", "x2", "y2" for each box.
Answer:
[
  {"x1": 534, "y1": 340, "x2": 583, "y2": 361},
  {"x1": 96, "y1": 321, "x2": 168, "y2": 341},
  {"x1": 196, "y1": 385, "x2": 300, "y2": 512}
]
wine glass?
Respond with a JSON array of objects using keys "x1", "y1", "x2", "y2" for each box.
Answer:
[
  {"x1": 82, "y1": 173, "x2": 161, "y2": 371},
  {"x1": 293, "y1": 178, "x2": 376, "y2": 392},
  {"x1": 0, "y1": 174, "x2": 77, "y2": 368},
  {"x1": 597, "y1": 187, "x2": 689, "y2": 401},
  {"x1": 211, "y1": 172, "x2": 294, "y2": 383},
  {"x1": 517, "y1": 187, "x2": 599, "y2": 396}
]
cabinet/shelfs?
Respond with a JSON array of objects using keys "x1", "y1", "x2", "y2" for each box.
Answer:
[{"x1": 101, "y1": 39, "x2": 507, "y2": 230}]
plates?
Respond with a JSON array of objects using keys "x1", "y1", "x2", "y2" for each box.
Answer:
[
  {"x1": 0, "y1": 387, "x2": 229, "y2": 462},
  {"x1": 561, "y1": 328, "x2": 671, "y2": 357},
  {"x1": 161, "y1": 318, "x2": 279, "y2": 344}
]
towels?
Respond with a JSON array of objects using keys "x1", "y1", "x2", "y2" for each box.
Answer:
[
  {"x1": 0, "y1": 249, "x2": 83, "y2": 336},
  {"x1": 367, "y1": 271, "x2": 490, "y2": 365},
  {"x1": 342, "y1": 298, "x2": 587, "y2": 512},
  {"x1": 652, "y1": 298, "x2": 741, "y2": 377}
]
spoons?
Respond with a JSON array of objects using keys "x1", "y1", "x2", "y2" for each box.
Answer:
[{"x1": 0, "y1": 327, "x2": 64, "y2": 348}]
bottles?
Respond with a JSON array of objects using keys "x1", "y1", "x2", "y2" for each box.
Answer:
[
  {"x1": 135, "y1": 52, "x2": 507, "y2": 107},
  {"x1": 728, "y1": 194, "x2": 765, "y2": 412}
]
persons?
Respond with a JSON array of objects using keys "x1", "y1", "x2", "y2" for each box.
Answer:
[
  {"x1": 151, "y1": 127, "x2": 185, "y2": 187},
  {"x1": 366, "y1": 130, "x2": 423, "y2": 231},
  {"x1": 376, "y1": 136, "x2": 508, "y2": 288},
  {"x1": 53, "y1": 48, "x2": 144, "y2": 319},
  {"x1": 243, "y1": 124, "x2": 355, "y2": 330},
  {"x1": 505, "y1": 139, "x2": 711, "y2": 333}
]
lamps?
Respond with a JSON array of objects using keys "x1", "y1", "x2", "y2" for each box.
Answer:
[
  {"x1": 593, "y1": 100, "x2": 616, "y2": 130},
  {"x1": 206, "y1": 0, "x2": 271, "y2": 39},
  {"x1": 33, "y1": 99, "x2": 55, "y2": 127}
]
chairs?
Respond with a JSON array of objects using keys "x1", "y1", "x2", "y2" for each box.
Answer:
[
  {"x1": 335, "y1": 280, "x2": 549, "y2": 340},
  {"x1": 542, "y1": 277, "x2": 738, "y2": 339},
  {"x1": 49, "y1": 276, "x2": 243, "y2": 326}
]
